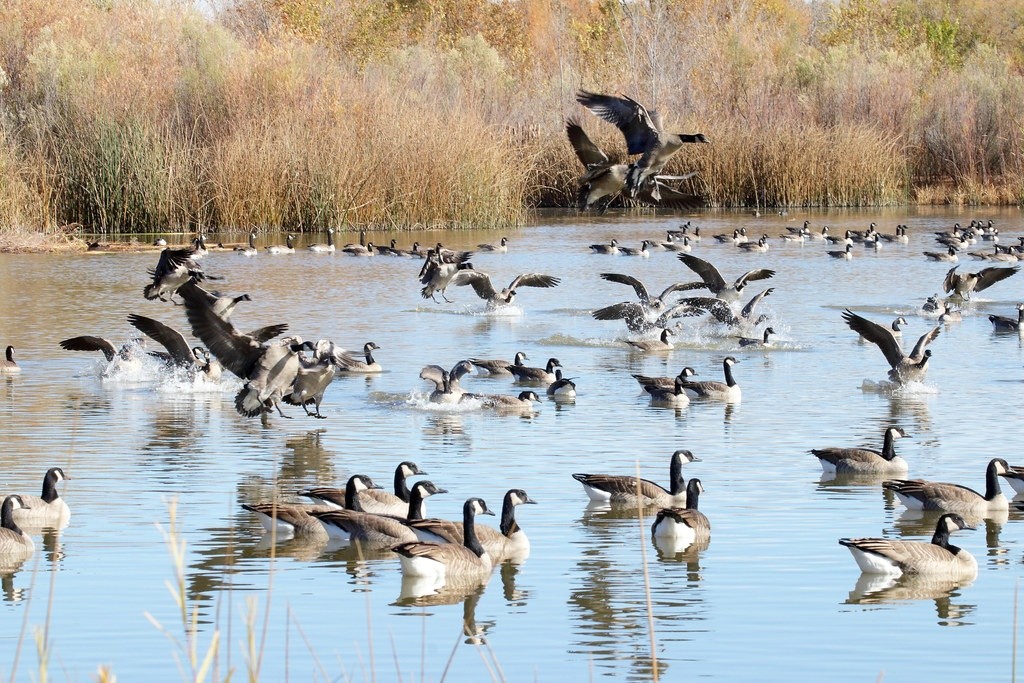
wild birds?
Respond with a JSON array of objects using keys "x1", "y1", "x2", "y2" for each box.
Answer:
[
  {"x1": 238, "y1": 474, "x2": 385, "y2": 539},
  {"x1": 381, "y1": 498, "x2": 497, "y2": 576},
  {"x1": 21, "y1": 527, "x2": 66, "y2": 562},
  {"x1": 571, "y1": 449, "x2": 704, "y2": 507},
  {"x1": 0, "y1": 495, "x2": 35, "y2": 552},
  {"x1": 810, "y1": 429, "x2": 913, "y2": 474},
  {"x1": 564, "y1": 87, "x2": 712, "y2": 212},
  {"x1": 0, "y1": 467, "x2": 71, "y2": 529},
  {"x1": 59, "y1": 220, "x2": 1024, "y2": 418},
  {"x1": 309, "y1": 459, "x2": 429, "y2": 518},
  {"x1": 837, "y1": 512, "x2": 979, "y2": 578},
  {"x1": 245, "y1": 529, "x2": 530, "y2": 645},
  {"x1": 0, "y1": 345, "x2": 21, "y2": 370},
  {"x1": 651, "y1": 478, "x2": 711, "y2": 544},
  {"x1": 840, "y1": 503, "x2": 1024, "y2": 626},
  {"x1": 840, "y1": 308, "x2": 941, "y2": 383},
  {"x1": 883, "y1": 457, "x2": 1024, "y2": 527},
  {"x1": 0, "y1": 552, "x2": 30, "y2": 605},
  {"x1": 306, "y1": 481, "x2": 448, "y2": 543},
  {"x1": 408, "y1": 488, "x2": 537, "y2": 562}
]
